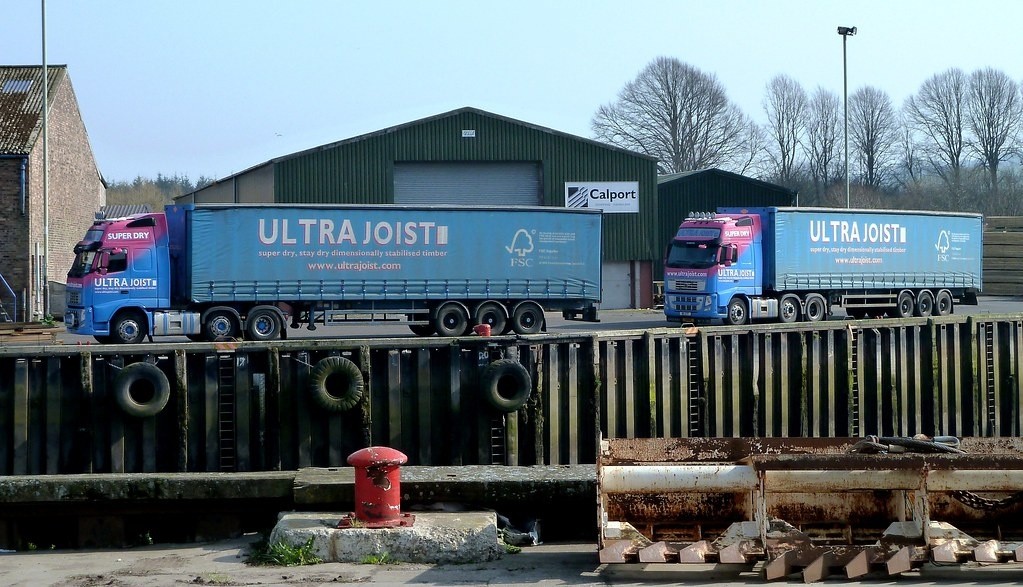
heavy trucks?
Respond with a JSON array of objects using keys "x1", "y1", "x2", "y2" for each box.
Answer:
[
  {"x1": 664, "y1": 205, "x2": 983, "y2": 325},
  {"x1": 64, "y1": 201, "x2": 602, "y2": 343}
]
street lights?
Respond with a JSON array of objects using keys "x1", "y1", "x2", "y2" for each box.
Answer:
[{"x1": 838, "y1": 27, "x2": 858, "y2": 207}]
